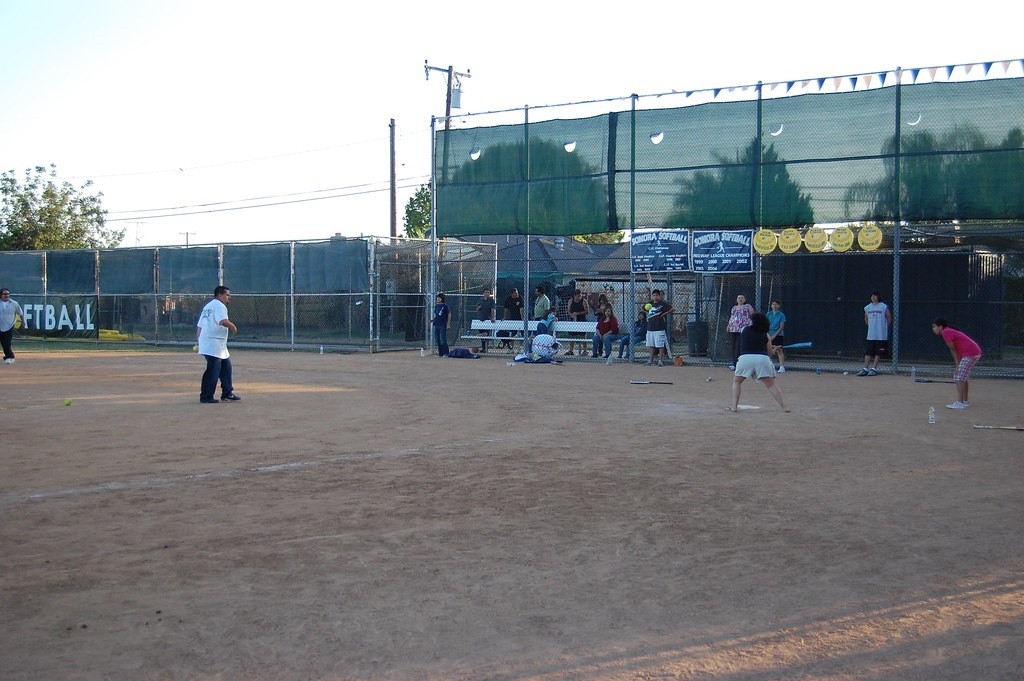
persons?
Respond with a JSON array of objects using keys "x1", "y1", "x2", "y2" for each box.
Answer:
[
  {"x1": 765, "y1": 300, "x2": 786, "y2": 373},
  {"x1": 536, "y1": 306, "x2": 558, "y2": 336},
  {"x1": 476, "y1": 289, "x2": 497, "y2": 353},
  {"x1": 196, "y1": 286, "x2": 241, "y2": 403},
  {"x1": 591, "y1": 306, "x2": 620, "y2": 359},
  {"x1": 728, "y1": 312, "x2": 791, "y2": 414},
  {"x1": 433, "y1": 293, "x2": 451, "y2": 358},
  {"x1": 503, "y1": 287, "x2": 524, "y2": 346},
  {"x1": 642, "y1": 289, "x2": 675, "y2": 367},
  {"x1": 931, "y1": 316, "x2": 983, "y2": 409},
  {"x1": 726, "y1": 293, "x2": 754, "y2": 372},
  {"x1": 594, "y1": 294, "x2": 613, "y2": 357},
  {"x1": 564, "y1": 289, "x2": 590, "y2": 356},
  {"x1": 616, "y1": 311, "x2": 647, "y2": 360},
  {"x1": 0, "y1": 288, "x2": 27, "y2": 365},
  {"x1": 856, "y1": 290, "x2": 892, "y2": 376},
  {"x1": 533, "y1": 285, "x2": 550, "y2": 335}
]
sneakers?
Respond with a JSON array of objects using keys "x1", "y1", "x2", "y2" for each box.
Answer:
[
  {"x1": 856, "y1": 368, "x2": 878, "y2": 376},
  {"x1": 946, "y1": 401, "x2": 969, "y2": 409},
  {"x1": 658, "y1": 360, "x2": 663, "y2": 366},
  {"x1": 221, "y1": 393, "x2": 241, "y2": 402},
  {"x1": 645, "y1": 360, "x2": 653, "y2": 364},
  {"x1": 200, "y1": 398, "x2": 219, "y2": 404},
  {"x1": 778, "y1": 368, "x2": 785, "y2": 372}
]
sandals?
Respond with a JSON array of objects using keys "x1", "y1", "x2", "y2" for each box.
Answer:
[
  {"x1": 581, "y1": 350, "x2": 589, "y2": 356},
  {"x1": 564, "y1": 351, "x2": 574, "y2": 355}
]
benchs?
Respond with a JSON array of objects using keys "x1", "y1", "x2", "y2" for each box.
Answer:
[{"x1": 460, "y1": 320, "x2": 647, "y2": 356}]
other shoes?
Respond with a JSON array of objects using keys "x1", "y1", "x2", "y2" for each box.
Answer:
[
  {"x1": 4, "y1": 357, "x2": 15, "y2": 364},
  {"x1": 435, "y1": 355, "x2": 447, "y2": 358}
]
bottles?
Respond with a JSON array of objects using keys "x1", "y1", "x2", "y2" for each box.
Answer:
[
  {"x1": 420, "y1": 348, "x2": 424, "y2": 356},
  {"x1": 319, "y1": 345, "x2": 323, "y2": 354},
  {"x1": 608, "y1": 354, "x2": 612, "y2": 364},
  {"x1": 911, "y1": 366, "x2": 916, "y2": 382},
  {"x1": 929, "y1": 406, "x2": 936, "y2": 423}
]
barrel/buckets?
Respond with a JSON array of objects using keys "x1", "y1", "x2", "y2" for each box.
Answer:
[{"x1": 686, "y1": 321, "x2": 708, "y2": 356}]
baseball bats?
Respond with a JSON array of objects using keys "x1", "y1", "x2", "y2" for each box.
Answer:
[
  {"x1": 780, "y1": 341, "x2": 813, "y2": 349},
  {"x1": 915, "y1": 379, "x2": 954, "y2": 384},
  {"x1": 630, "y1": 379, "x2": 673, "y2": 384},
  {"x1": 973, "y1": 424, "x2": 1024, "y2": 432}
]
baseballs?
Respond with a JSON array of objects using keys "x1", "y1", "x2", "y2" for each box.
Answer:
[
  {"x1": 193, "y1": 345, "x2": 199, "y2": 352},
  {"x1": 844, "y1": 371, "x2": 849, "y2": 375},
  {"x1": 64, "y1": 398, "x2": 71, "y2": 406}
]
